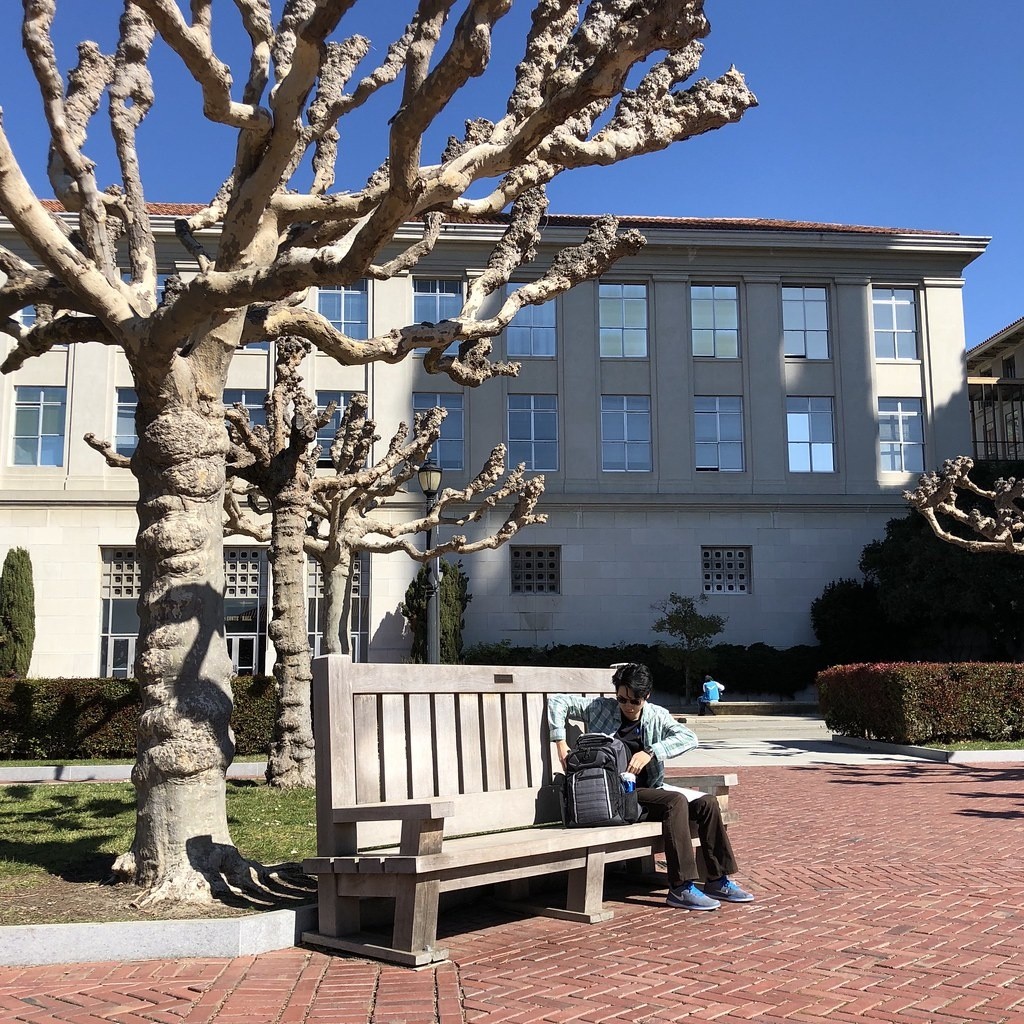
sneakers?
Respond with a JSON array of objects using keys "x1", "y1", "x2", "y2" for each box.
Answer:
[
  {"x1": 703, "y1": 876, "x2": 754, "y2": 901},
  {"x1": 666, "y1": 883, "x2": 721, "y2": 910}
]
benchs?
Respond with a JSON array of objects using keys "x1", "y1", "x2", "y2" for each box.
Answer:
[{"x1": 302, "y1": 655, "x2": 737, "y2": 965}]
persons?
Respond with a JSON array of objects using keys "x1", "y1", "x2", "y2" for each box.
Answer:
[
  {"x1": 545, "y1": 663, "x2": 755, "y2": 910},
  {"x1": 696, "y1": 675, "x2": 726, "y2": 715}
]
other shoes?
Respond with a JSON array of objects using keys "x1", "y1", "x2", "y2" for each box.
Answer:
[
  {"x1": 712, "y1": 713, "x2": 715, "y2": 715},
  {"x1": 698, "y1": 713, "x2": 705, "y2": 716}
]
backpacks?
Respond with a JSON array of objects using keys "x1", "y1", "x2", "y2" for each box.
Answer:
[{"x1": 559, "y1": 732, "x2": 649, "y2": 826}]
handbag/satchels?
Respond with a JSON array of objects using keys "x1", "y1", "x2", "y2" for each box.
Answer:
[{"x1": 533, "y1": 772, "x2": 565, "y2": 825}]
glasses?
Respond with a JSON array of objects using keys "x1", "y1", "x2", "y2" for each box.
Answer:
[{"x1": 616, "y1": 695, "x2": 641, "y2": 705}]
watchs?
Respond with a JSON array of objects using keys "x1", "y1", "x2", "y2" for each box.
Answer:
[{"x1": 645, "y1": 745, "x2": 655, "y2": 758}]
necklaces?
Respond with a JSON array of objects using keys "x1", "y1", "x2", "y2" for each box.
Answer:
[{"x1": 631, "y1": 717, "x2": 639, "y2": 720}]
